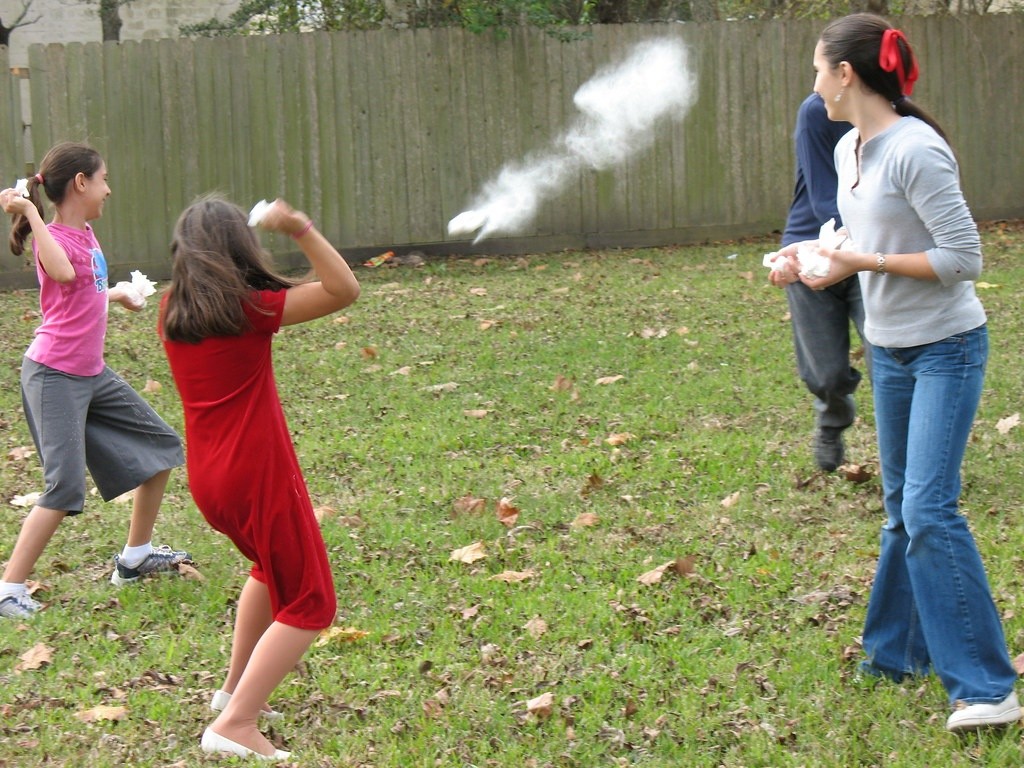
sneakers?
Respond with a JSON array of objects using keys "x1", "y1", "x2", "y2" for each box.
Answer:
[
  {"x1": 0, "y1": 591, "x2": 42, "y2": 620},
  {"x1": 111, "y1": 545, "x2": 192, "y2": 586}
]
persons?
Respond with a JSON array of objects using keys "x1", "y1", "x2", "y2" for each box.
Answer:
[
  {"x1": 781, "y1": 92, "x2": 856, "y2": 470},
  {"x1": 0, "y1": 143, "x2": 193, "y2": 619},
  {"x1": 159, "y1": 198, "x2": 359, "y2": 761},
  {"x1": 766, "y1": 15, "x2": 1023, "y2": 730}
]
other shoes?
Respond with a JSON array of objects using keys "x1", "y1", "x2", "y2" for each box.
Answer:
[
  {"x1": 210, "y1": 691, "x2": 282, "y2": 720},
  {"x1": 947, "y1": 691, "x2": 1022, "y2": 732},
  {"x1": 202, "y1": 728, "x2": 292, "y2": 762},
  {"x1": 816, "y1": 430, "x2": 842, "y2": 470}
]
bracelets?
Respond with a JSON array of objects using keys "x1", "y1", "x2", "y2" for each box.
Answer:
[
  {"x1": 876, "y1": 252, "x2": 885, "y2": 276},
  {"x1": 292, "y1": 221, "x2": 313, "y2": 241}
]
